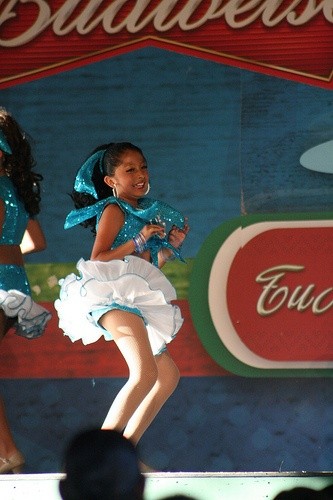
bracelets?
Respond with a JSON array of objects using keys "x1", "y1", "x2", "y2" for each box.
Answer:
[{"x1": 133, "y1": 232, "x2": 148, "y2": 255}]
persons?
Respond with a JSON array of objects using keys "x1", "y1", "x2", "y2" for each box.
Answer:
[
  {"x1": 53, "y1": 140, "x2": 190, "y2": 452},
  {"x1": 58, "y1": 426, "x2": 146, "y2": 500},
  {"x1": 0, "y1": 106, "x2": 50, "y2": 476}
]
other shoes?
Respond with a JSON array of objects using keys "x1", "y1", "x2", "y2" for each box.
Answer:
[{"x1": 0, "y1": 453, "x2": 25, "y2": 474}]
